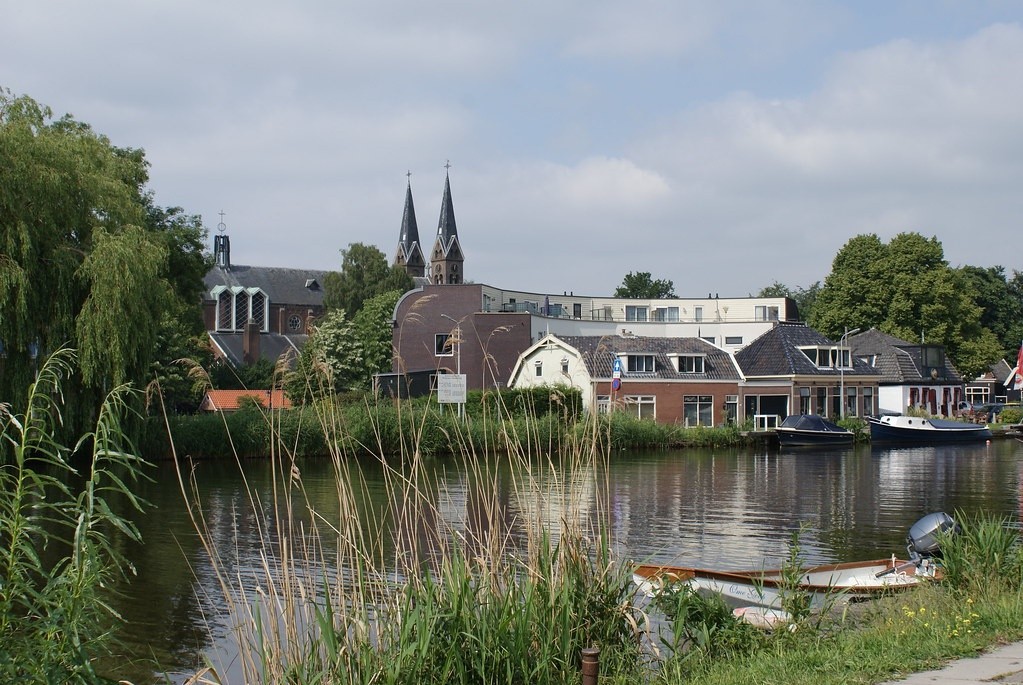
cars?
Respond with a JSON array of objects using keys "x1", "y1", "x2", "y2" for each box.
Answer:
[{"x1": 955, "y1": 399, "x2": 982, "y2": 418}]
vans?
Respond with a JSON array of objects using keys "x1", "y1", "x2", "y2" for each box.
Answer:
[{"x1": 969, "y1": 402, "x2": 1023, "y2": 423}]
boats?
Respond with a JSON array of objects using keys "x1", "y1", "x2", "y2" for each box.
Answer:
[
  {"x1": 865, "y1": 412, "x2": 993, "y2": 445},
  {"x1": 627, "y1": 512, "x2": 961, "y2": 640},
  {"x1": 773, "y1": 411, "x2": 856, "y2": 447}
]
